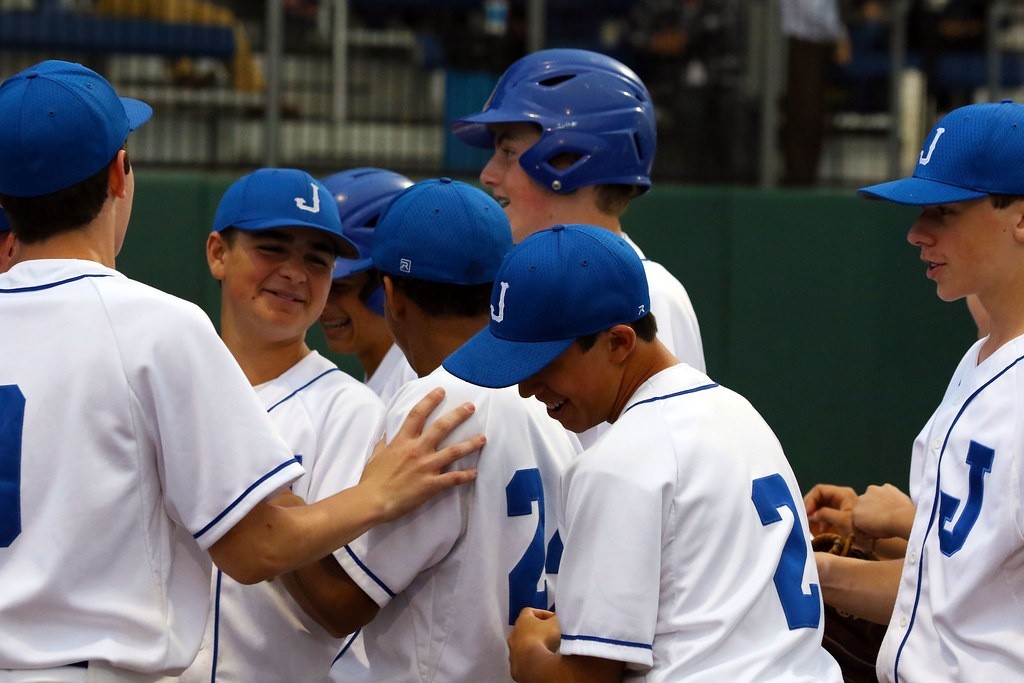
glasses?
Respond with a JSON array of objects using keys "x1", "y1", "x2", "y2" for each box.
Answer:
[{"x1": 325, "y1": 278, "x2": 368, "y2": 301}]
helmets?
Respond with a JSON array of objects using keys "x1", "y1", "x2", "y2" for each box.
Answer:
[
  {"x1": 319, "y1": 167, "x2": 417, "y2": 317},
  {"x1": 449, "y1": 48, "x2": 657, "y2": 199}
]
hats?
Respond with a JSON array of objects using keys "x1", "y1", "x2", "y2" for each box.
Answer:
[
  {"x1": 442, "y1": 224, "x2": 652, "y2": 389},
  {"x1": 0, "y1": 60, "x2": 154, "y2": 196},
  {"x1": 856, "y1": 99, "x2": 1024, "y2": 208},
  {"x1": 348, "y1": 178, "x2": 514, "y2": 285},
  {"x1": 212, "y1": 168, "x2": 361, "y2": 261}
]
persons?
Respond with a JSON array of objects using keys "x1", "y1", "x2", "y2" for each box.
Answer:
[
  {"x1": 421, "y1": 1, "x2": 851, "y2": 189},
  {"x1": 802, "y1": 100, "x2": 1024, "y2": 683},
  {"x1": 0, "y1": 48, "x2": 842, "y2": 683}
]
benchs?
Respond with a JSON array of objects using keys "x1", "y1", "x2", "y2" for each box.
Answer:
[{"x1": 0, "y1": 11, "x2": 234, "y2": 174}]
